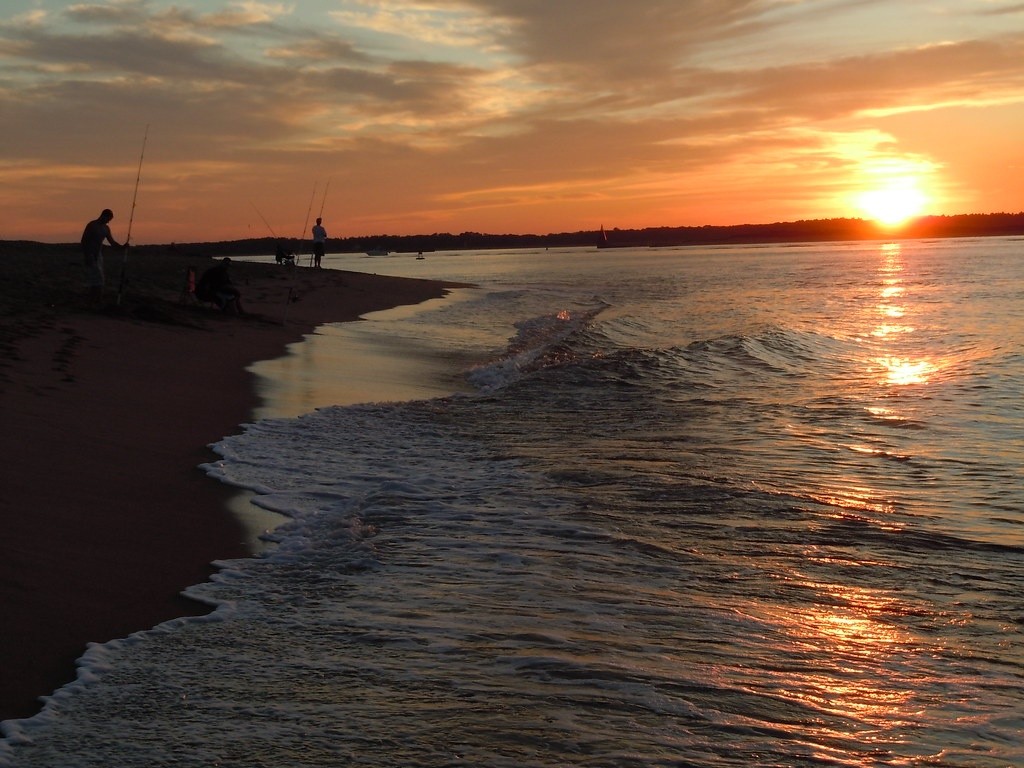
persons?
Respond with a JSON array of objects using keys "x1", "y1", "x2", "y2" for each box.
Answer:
[
  {"x1": 196, "y1": 258, "x2": 248, "y2": 316},
  {"x1": 312, "y1": 218, "x2": 327, "y2": 269},
  {"x1": 80, "y1": 210, "x2": 128, "y2": 309}
]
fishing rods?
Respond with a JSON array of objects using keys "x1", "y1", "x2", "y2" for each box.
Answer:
[
  {"x1": 114, "y1": 125, "x2": 152, "y2": 303},
  {"x1": 248, "y1": 175, "x2": 332, "y2": 325}
]
